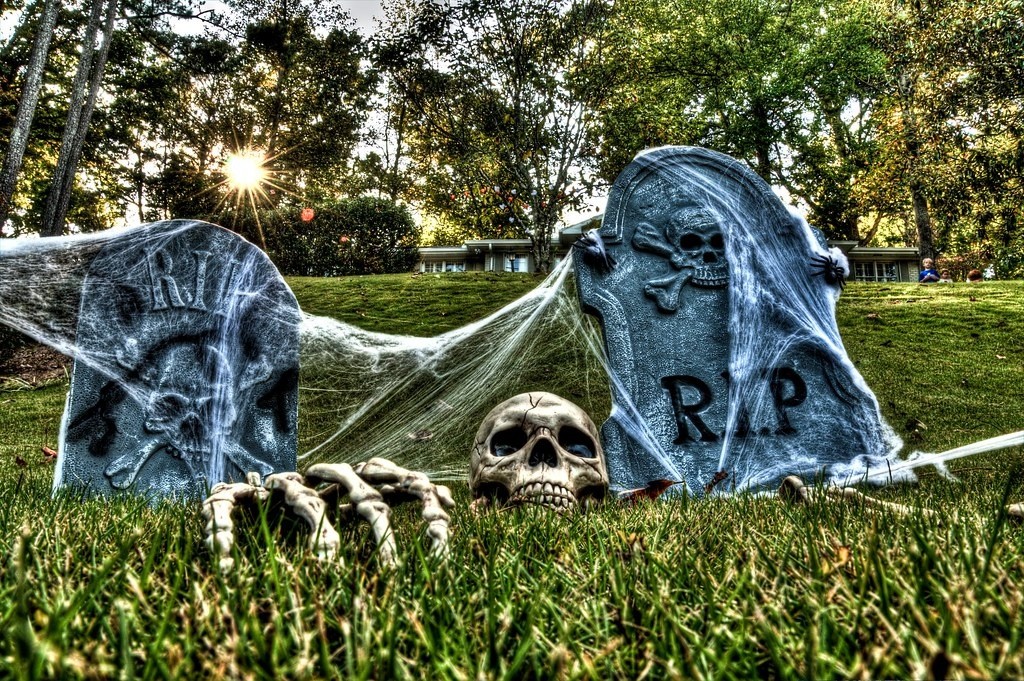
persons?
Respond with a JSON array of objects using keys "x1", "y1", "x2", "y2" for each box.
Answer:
[
  {"x1": 919, "y1": 258, "x2": 940, "y2": 283},
  {"x1": 966, "y1": 270, "x2": 984, "y2": 283},
  {"x1": 940, "y1": 269, "x2": 953, "y2": 283}
]
